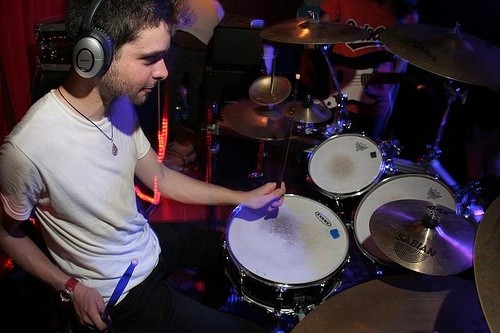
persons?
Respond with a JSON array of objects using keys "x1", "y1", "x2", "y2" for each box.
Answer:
[
  {"x1": 153, "y1": 0, "x2": 421, "y2": 136},
  {"x1": 0, "y1": 0, "x2": 286, "y2": 333}
]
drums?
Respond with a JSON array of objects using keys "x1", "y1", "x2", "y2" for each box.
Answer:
[
  {"x1": 352, "y1": 172, "x2": 461, "y2": 271},
  {"x1": 306, "y1": 132, "x2": 386, "y2": 235},
  {"x1": 222, "y1": 193, "x2": 352, "y2": 320}
]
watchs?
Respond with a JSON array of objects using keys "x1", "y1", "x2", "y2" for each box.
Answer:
[{"x1": 59, "y1": 277, "x2": 79, "y2": 302}]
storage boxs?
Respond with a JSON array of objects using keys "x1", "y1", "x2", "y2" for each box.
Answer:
[{"x1": 168, "y1": 15, "x2": 264, "y2": 126}]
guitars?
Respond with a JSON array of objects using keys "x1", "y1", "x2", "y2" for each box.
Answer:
[{"x1": 311, "y1": 64, "x2": 415, "y2": 110}]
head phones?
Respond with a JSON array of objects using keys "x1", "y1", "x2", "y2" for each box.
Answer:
[{"x1": 70, "y1": 0, "x2": 114, "y2": 78}]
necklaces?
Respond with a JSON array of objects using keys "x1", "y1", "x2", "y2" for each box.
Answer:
[{"x1": 58, "y1": 87, "x2": 118, "y2": 156}]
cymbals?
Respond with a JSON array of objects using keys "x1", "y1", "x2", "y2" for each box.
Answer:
[
  {"x1": 369, "y1": 199, "x2": 480, "y2": 278},
  {"x1": 223, "y1": 99, "x2": 297, "y2": 140},
  {"x1": 288, "y1": 279, "x2": 492, "y2": 333},
  {"x1": 248, "y1": 74, "x2": 291, "y2": 106},
  {"x1": 281, "y1": 101, "x2": 333, "y2": 124},
  {"x1": 258, "y1": 19, "x2": 371, "y2": 45},
  {"x1": 379, "y1": 23, "x2": 500, "y2": 88},
  {"x1": 472, "y1": 196, "x2": 500, "y2": 333}
]
